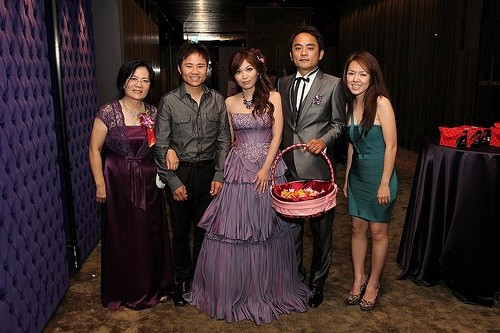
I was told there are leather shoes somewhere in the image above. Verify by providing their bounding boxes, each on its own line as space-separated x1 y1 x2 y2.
307 284 324 308
173 280 189 307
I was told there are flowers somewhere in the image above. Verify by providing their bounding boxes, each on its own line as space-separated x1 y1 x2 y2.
138 110 156 128
312 96 322 105
248 48 268 64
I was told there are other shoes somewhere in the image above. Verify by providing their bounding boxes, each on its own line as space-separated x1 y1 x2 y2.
159 295 169 303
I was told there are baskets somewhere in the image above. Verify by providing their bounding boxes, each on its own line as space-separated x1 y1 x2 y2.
270 143 337 218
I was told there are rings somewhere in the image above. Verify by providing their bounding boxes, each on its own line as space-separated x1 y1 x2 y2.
313 148 317 151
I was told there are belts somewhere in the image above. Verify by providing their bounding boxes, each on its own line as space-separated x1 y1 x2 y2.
178 160 210 169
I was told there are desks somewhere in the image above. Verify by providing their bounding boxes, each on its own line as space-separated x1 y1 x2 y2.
396 133 500 308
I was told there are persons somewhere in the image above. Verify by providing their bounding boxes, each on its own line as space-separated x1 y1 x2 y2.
276 27 345 310
89 59 179 310
155 44 231 306
182 49 315 326
343 50 399 310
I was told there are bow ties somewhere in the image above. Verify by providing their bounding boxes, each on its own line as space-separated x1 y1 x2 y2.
295 67 320 111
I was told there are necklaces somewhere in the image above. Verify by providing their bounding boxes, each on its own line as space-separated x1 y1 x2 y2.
241 91 255 109
122 99 145 127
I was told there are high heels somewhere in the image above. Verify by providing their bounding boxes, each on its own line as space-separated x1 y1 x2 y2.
346 273 368 304
360 279 380 311
